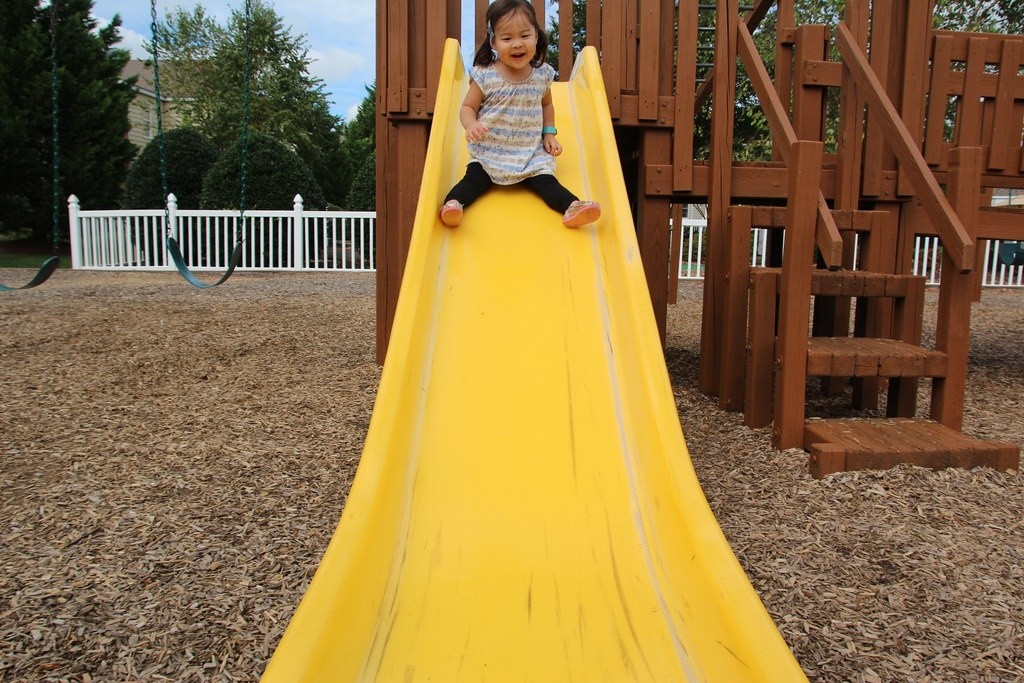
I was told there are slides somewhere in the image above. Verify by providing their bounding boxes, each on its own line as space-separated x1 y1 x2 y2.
257 37 815 679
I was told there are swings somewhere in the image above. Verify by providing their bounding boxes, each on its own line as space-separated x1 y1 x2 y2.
0 0 64 292
149 0 252 291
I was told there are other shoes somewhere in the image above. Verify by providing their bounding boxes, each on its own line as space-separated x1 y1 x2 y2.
563 201 601 228
439 199 462 227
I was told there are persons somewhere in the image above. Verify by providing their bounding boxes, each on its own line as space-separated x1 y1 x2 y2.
441 0 601 228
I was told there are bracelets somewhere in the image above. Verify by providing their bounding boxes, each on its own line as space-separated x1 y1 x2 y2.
543 127 558 136
